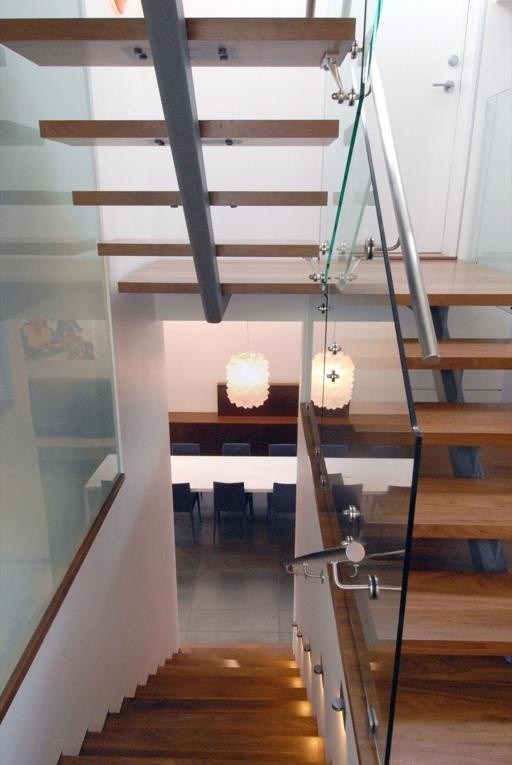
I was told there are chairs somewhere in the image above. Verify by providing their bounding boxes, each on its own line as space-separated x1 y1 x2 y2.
328 472 363 538
172 443 296 546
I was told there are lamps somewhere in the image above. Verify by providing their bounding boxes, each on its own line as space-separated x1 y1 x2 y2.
225 321 270 410
311 322 356 411
